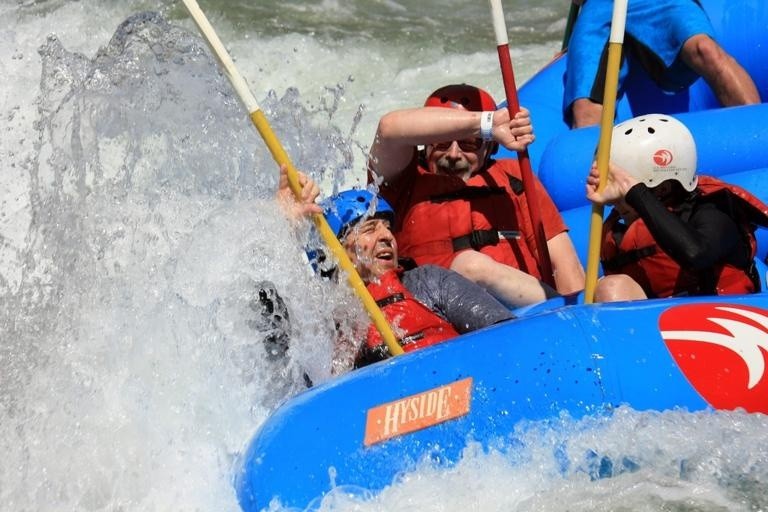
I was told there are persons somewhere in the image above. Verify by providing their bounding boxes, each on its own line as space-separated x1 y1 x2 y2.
563 0 762 130
585 113 768 304
368 84 586 311
259 165 517 388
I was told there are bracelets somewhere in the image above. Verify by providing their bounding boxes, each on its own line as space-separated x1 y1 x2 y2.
480 111 494 142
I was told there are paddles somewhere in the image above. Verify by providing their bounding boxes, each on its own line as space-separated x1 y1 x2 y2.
184 0 405 356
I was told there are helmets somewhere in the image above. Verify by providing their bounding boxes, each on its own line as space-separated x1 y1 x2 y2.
610 113 699 191
424 84 500 156
306 189 394 276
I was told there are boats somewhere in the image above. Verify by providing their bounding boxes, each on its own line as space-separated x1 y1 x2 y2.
233 0 768 512
